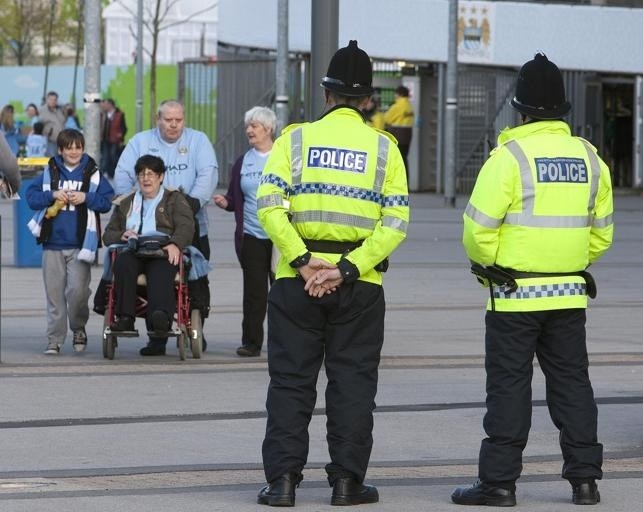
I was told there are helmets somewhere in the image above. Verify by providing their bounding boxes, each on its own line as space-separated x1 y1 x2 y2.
509 52 573 119
319 40 374 97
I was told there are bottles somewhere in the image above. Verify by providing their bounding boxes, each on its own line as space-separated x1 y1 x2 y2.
44 197 65 220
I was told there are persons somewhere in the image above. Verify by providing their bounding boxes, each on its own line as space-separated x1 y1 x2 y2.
102 153 196 333
383 85 415 194
212 106 279 357
0 91 128 201
450 50 614 508
364 94 384 130
24 129 115 355
254 39 410 506
112 98 219 357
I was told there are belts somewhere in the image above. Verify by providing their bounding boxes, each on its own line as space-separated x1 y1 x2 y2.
502 269 582 279
302 239 356 253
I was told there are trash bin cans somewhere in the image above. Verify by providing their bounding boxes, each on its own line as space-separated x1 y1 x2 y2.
10 171 45 268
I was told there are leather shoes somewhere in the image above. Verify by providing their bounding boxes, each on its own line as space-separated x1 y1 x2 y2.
112 320 167 356
572 481 600 504
237 344 260 356
331 478 378 505
258 477 295 506
452 480 516 506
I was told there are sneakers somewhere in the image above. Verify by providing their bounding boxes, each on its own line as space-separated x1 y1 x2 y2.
74 329 86 352
45 345 60 354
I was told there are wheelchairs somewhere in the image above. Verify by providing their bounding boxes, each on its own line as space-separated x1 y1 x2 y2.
96 238 207 362
